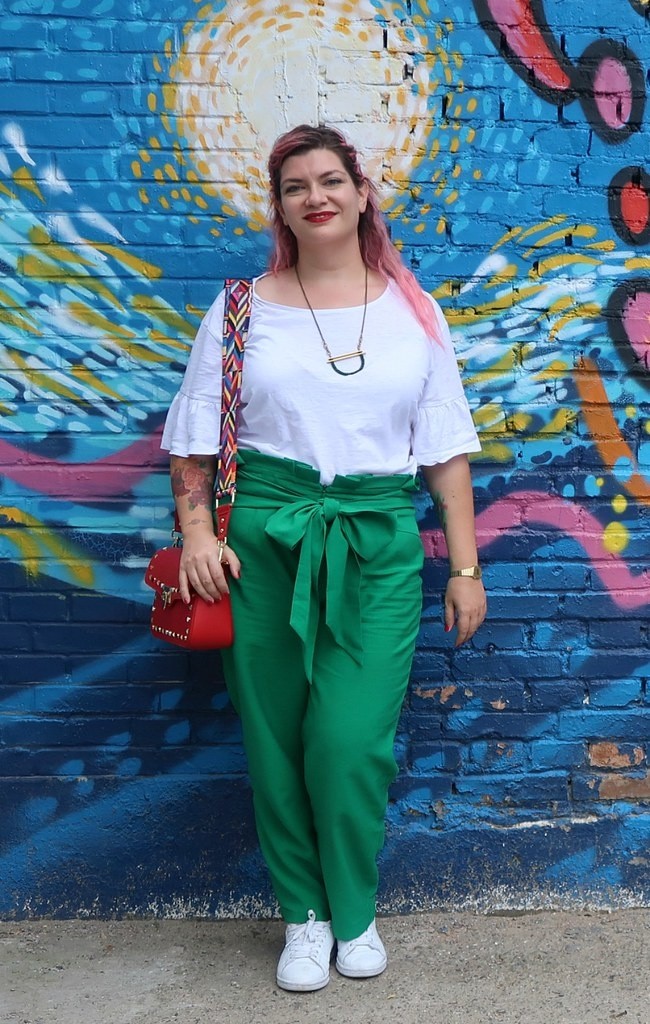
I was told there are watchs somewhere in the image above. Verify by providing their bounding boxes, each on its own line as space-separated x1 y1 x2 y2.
449 565 482 579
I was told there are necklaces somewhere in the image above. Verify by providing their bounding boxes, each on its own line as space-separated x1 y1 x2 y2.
293 257 368 377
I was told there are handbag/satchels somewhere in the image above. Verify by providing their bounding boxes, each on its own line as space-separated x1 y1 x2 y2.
144 545 233 649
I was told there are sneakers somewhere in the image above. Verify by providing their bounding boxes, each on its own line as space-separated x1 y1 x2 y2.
335 917 387 977
276 908 335 992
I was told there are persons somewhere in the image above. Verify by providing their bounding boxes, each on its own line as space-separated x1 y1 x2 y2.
167 126 489 991
